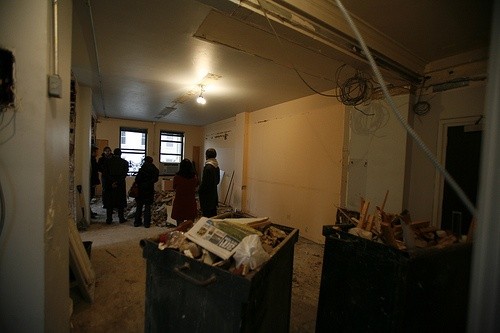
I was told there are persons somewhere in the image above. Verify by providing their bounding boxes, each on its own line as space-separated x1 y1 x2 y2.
98 147 114 208
198 148 220 218
171 159 200 227
106 149 129 224
90 147 101 219
134 156 159 228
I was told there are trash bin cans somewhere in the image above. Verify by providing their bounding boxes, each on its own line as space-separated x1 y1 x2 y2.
314 190 472 333
140 211 299 333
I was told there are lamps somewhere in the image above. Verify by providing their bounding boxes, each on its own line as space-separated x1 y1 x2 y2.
196 86 206 104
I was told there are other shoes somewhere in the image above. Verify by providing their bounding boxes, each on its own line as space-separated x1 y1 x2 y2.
144 223 151 228
106 220 112 224
134 223 143 227
120 219 127 223
91 213 97 219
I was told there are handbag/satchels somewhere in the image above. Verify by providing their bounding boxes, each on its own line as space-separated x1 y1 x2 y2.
129 182 136 197
